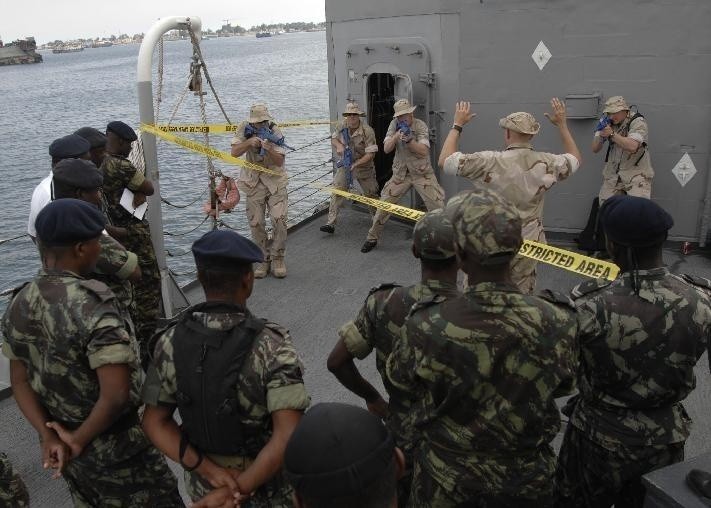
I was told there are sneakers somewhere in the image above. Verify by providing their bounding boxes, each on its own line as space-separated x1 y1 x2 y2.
270 256 287 277
361 239 378 253
254 259 273 278
320 224 336 233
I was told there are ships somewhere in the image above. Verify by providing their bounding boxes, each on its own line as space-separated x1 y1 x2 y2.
0 37 113 65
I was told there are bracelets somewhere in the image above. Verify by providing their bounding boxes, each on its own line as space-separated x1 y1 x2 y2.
451 125 462 133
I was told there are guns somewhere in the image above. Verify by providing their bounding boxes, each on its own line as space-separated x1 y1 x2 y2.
244 124 296 156
396 120 410 136
336 128 353 195
594 117 610 133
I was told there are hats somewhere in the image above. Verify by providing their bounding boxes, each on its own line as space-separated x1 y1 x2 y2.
191 230 265 271
413 188 523 265
500 112 542 136
342 102 366 117
603 95 631 113
246 105 274 124
598 195 674 248
282 404 397 499
35 122 138 245
393 99 417 118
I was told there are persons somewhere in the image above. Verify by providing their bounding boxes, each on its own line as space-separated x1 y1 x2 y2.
231 102 289 279
385 187 579 507
1 197 182 507
29 121 163 326
361 99 444 251
437 97 582 296
327 207 464 508
285 403 406 508
319 104 379 234
553 194 710 508
140 229 312 507
593 96 655 206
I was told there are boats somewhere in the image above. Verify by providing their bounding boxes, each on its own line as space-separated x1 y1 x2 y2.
255 30 272 37
